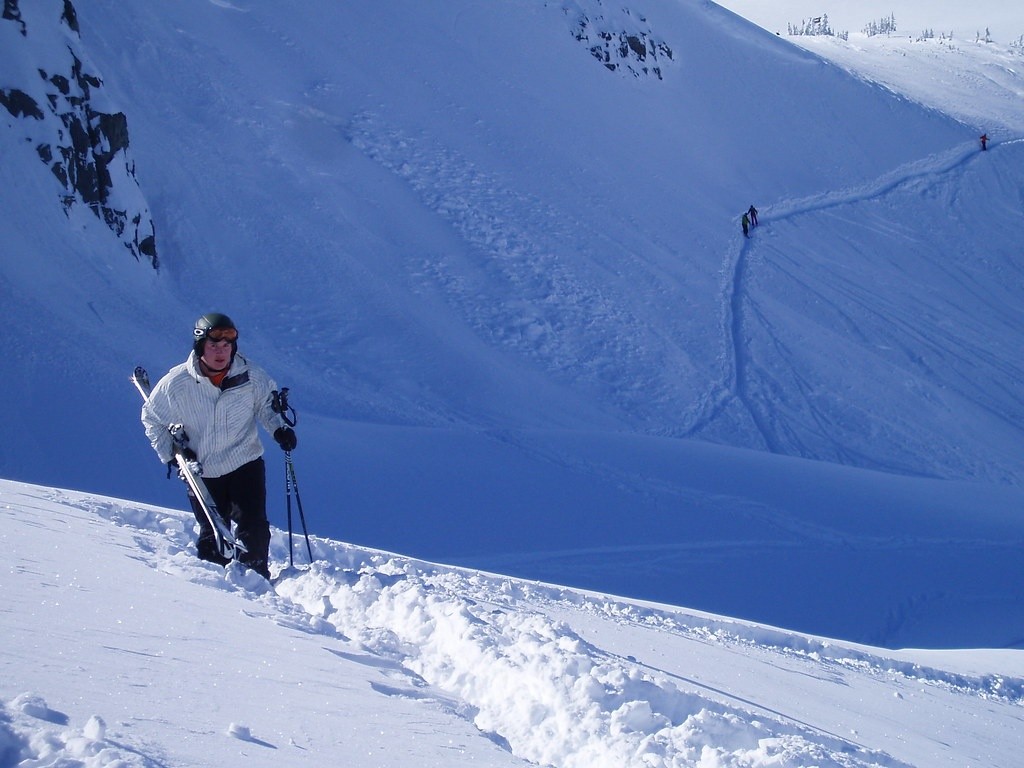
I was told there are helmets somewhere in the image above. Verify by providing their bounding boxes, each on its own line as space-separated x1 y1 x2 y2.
193 312 238 356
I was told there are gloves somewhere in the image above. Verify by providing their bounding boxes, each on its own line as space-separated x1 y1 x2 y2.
170 448 202 478
274 425 298 451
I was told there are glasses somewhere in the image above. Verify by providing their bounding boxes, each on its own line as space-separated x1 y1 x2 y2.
206 326 240 343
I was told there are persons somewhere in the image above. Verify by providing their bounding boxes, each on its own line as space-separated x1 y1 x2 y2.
742 205 758 236
980 134 990 150
141 314 297 579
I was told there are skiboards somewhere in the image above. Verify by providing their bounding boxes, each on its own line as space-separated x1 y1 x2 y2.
129 366 249 559
